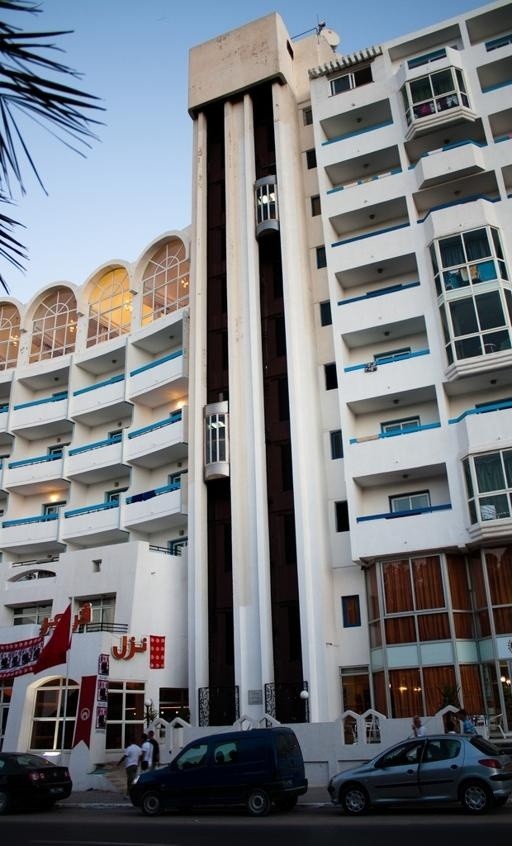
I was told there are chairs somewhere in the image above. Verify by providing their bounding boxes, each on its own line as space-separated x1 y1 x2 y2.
484 714 507 740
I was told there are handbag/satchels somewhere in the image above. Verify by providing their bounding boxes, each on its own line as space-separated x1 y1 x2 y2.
141 760 149 771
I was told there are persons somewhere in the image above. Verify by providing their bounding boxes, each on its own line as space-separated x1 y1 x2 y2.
447 721 457 733
117 730 160 800
413 715 424 736
456 710 476 734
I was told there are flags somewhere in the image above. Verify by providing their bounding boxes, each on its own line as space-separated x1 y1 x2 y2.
34 603 73 675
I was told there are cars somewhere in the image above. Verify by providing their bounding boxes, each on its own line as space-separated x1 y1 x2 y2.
0 752 72 816
327 734 512 816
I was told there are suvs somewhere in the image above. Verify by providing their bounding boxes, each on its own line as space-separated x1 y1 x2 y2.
128 727 308 816
